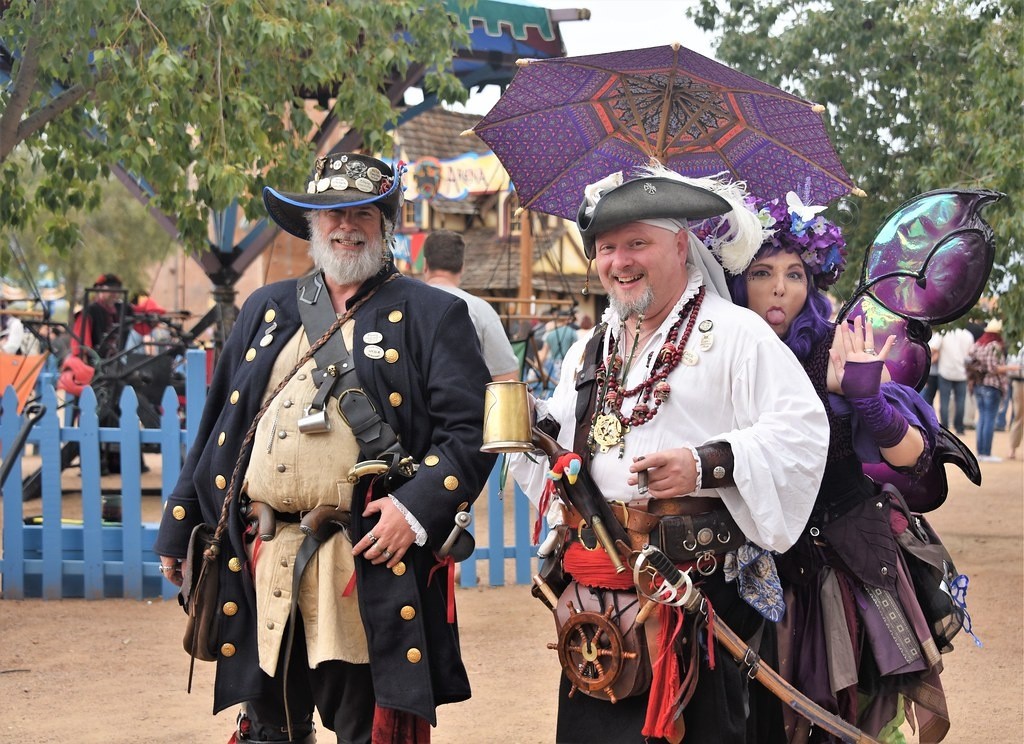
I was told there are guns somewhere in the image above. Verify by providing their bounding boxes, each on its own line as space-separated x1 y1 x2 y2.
530 426 625 575
299 505 351 543
238 502 277 542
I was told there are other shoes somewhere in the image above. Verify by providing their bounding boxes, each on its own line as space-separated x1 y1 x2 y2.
1008 449 1015 459
956 430 964 436
963 425 976 430
994 425 1006 431
978 455 1002 462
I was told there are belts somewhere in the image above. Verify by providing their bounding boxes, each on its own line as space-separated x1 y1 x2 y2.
272 510 343 742
560 497 753 564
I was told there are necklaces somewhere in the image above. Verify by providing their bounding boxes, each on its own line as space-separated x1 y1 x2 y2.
626 323 664 353
587 282 706 459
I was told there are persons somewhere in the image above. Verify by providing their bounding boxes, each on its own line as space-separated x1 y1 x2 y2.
938 327 974 432
419 229 520 383
537 313 579 393
155 151 499 744
919 328 942 403
498 152 830 744
970 314 1020 461
1008 345 1024 456
71 272 136 474
576 314 593 337
694 188 1005 744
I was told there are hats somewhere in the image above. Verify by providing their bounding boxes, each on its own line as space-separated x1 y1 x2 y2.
93 275 122 288
576 177 733 260
263 152 407 240
984 319 1004 333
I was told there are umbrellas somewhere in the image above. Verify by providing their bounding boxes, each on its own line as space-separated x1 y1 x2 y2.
460 41 868 253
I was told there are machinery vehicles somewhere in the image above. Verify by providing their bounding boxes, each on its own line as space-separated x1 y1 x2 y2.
1 286 218 479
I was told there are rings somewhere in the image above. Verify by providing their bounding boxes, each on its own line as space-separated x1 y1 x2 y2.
383 550 392 559
391 556 400 565
374 542 384 554
367 532 377 543
864 348 875 354
158 565 175 574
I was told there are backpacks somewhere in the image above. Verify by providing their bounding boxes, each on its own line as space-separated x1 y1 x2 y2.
965 344 996 390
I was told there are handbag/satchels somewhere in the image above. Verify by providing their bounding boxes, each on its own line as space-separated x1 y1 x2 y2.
896 512 961 621
177 524 225 661
554 582 650 699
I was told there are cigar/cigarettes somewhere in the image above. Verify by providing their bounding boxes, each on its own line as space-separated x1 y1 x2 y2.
637 456 648 495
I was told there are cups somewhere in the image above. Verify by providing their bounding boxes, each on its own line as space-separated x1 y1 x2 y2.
479 380 537 455
